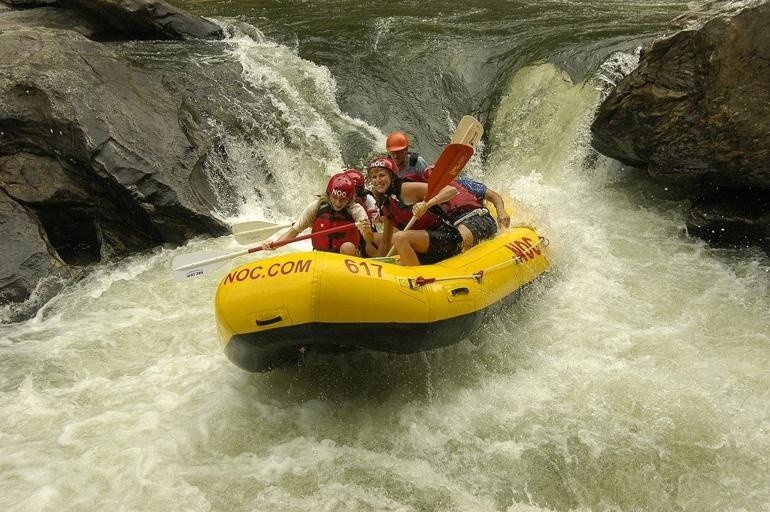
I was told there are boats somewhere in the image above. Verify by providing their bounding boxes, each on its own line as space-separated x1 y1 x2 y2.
214 194 551 374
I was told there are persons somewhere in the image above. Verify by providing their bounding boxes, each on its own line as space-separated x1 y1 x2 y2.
424 164 510 253
372 133 429 202
368 159 464 267
262 174 374 258
343 168 382 256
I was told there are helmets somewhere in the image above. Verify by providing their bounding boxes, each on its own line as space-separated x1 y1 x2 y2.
386 134 408 152
368 157 399 176
424 165 436 179
326 170 364 200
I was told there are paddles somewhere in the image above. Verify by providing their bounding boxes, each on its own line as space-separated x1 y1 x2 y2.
232 214 376 245
451 115 484 148
385 143 473 258
172 221 362 282
